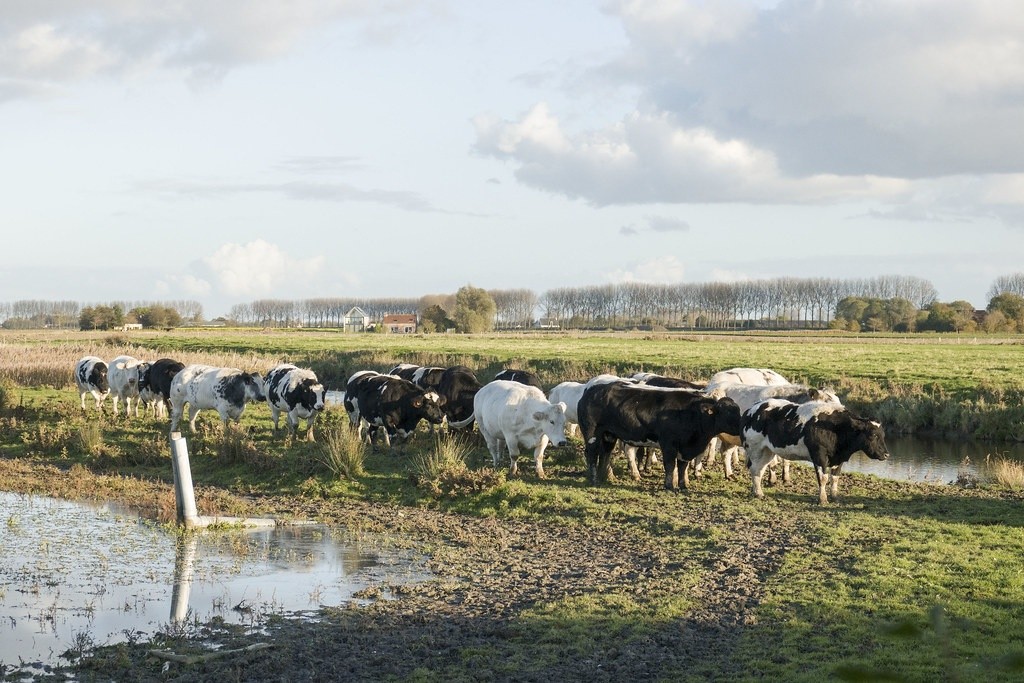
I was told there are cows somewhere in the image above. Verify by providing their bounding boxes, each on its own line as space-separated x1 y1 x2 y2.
75 355 891 506
